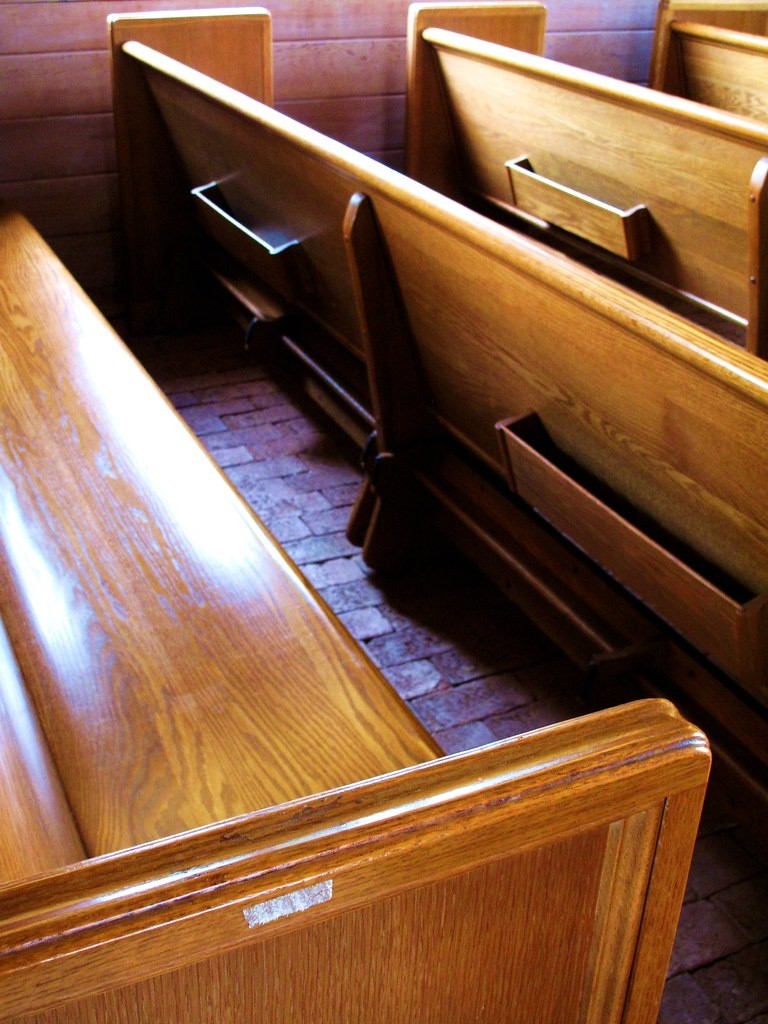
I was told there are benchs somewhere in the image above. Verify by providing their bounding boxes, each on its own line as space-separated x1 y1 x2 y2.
103 7 768 853
647 0 768 124
0 215 715 1024
405 2 768 363
